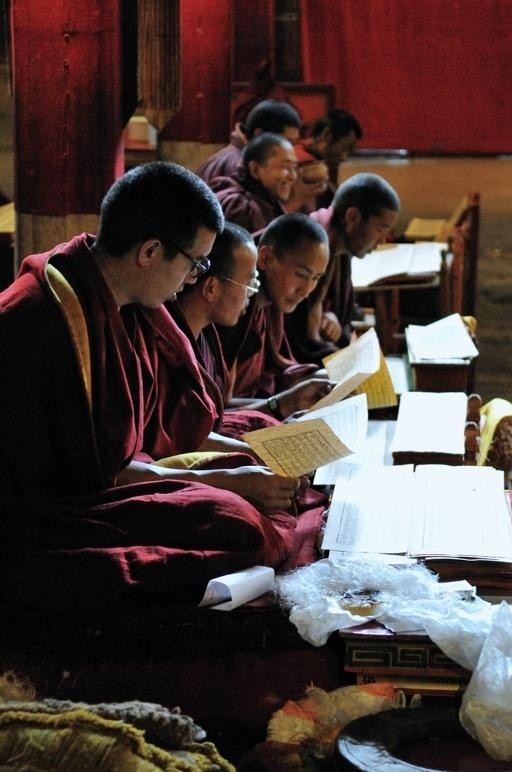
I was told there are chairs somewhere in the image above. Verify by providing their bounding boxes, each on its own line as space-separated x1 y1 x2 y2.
399 190 480 392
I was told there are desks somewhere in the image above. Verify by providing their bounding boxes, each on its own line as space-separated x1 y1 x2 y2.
351 241 439 352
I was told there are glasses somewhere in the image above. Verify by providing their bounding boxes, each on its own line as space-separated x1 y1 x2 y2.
180 250 211 279
224 270 261 298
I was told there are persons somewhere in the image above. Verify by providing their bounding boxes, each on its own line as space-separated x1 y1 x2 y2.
166 171 401 452
1 162 301 628
194 99 364 234
